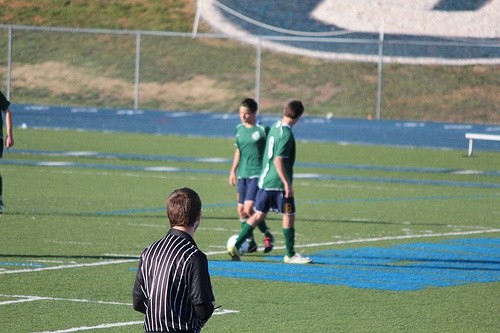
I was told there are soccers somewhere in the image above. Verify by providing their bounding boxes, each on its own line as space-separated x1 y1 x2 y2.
227 234 249 255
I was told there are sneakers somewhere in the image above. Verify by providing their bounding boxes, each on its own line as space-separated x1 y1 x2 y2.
228 245 240 260
263 236 273 253
284 249 312 264
239 240 257 253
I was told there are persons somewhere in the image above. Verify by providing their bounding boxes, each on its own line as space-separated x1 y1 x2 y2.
0 89 15 215
132 187 215 333
226 100 313 264
229 98 274 254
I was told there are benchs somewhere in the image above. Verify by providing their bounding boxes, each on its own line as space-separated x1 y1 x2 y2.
465 133 500 157
